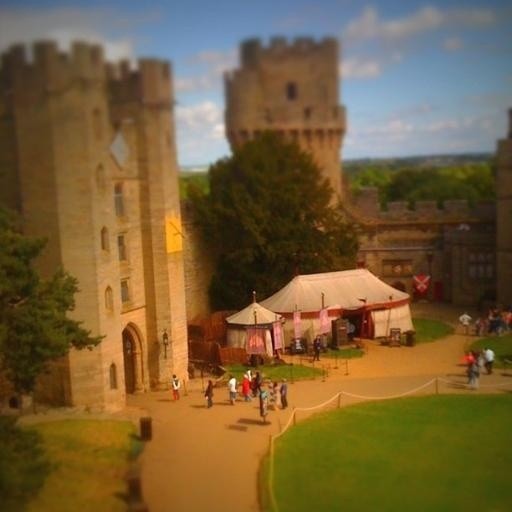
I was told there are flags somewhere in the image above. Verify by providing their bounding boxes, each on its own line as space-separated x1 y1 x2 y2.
292 309 302 338
318 305 332 334
270 320 284 349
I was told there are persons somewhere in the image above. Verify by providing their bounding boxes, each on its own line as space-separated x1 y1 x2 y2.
483 347 495 374
458 311 473 334
243 375 252 401
465 349 483 389
228 375 237 405
475 306 512 336
267 378 287 409
313 335 321 360
205 380 213 408
172 374 181 401
253 372 263 397
247 369 253 387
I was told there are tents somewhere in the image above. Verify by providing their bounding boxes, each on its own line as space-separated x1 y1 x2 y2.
225 291 287 363
258 268 417 352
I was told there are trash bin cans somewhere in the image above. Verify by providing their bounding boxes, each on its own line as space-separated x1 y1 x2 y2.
251 354 259 367
127 475 141 501
140 417 152 441
406 330 416 346
